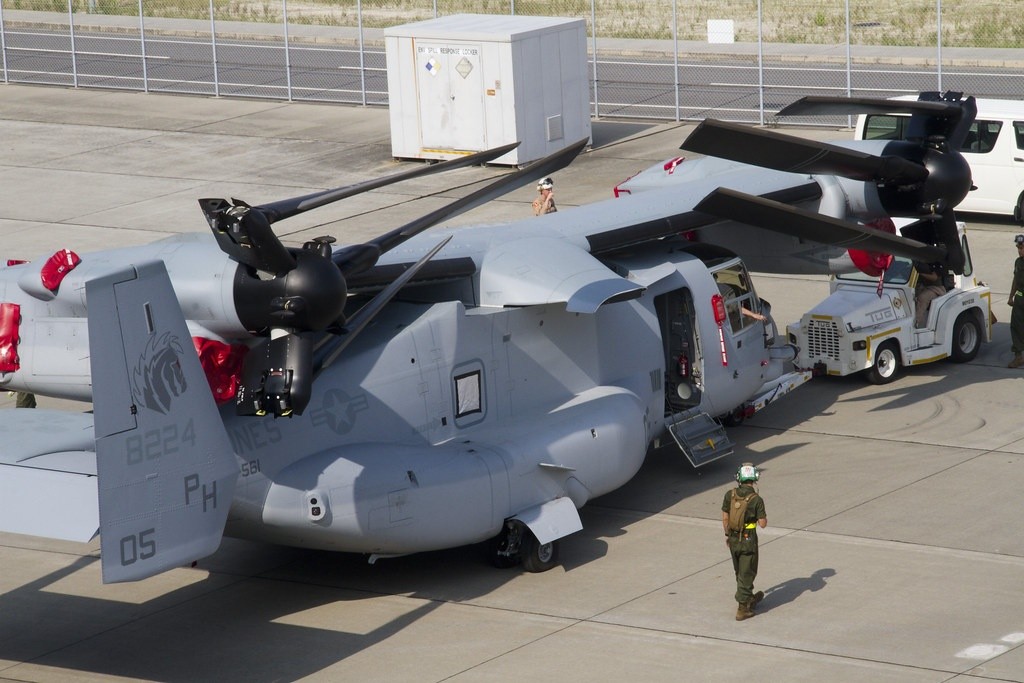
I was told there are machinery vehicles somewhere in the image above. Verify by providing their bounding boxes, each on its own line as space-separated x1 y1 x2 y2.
785 222 996 387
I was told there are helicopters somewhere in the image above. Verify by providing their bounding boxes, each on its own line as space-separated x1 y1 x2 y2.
607 81 979 273
0 135 775 585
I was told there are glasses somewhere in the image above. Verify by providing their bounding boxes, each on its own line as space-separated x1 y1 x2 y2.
542 178 553 185
1014 235 1024 243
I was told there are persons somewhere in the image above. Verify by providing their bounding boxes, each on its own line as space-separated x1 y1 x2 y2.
721 463 768 622
1008 235 1024 369
914 262 947 327
711 273 768 331
532 177 558 216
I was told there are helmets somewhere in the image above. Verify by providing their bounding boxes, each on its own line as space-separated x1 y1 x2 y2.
537 178 553 190
735 462 760 484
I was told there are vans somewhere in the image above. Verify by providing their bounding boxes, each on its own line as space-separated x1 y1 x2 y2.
854 94 1024 225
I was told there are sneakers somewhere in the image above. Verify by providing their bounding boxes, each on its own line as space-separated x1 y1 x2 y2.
1008 356 1024 368
736 608 756 620
751 591 764 609
